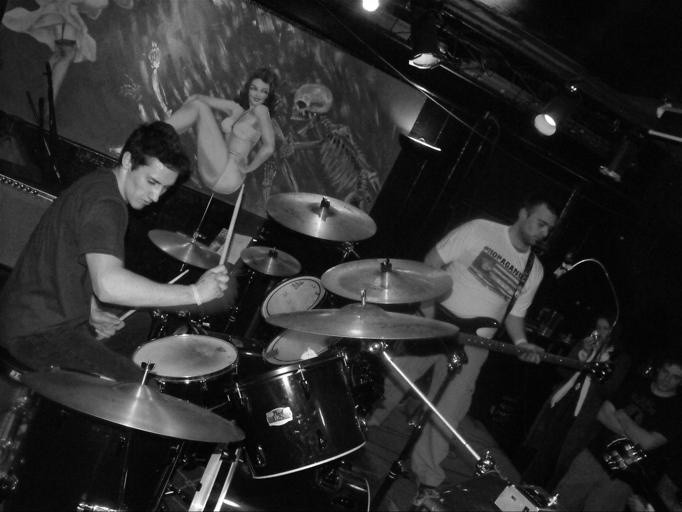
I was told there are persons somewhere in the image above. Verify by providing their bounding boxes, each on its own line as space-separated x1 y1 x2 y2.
1 119 234 391
156 70 276 196
350 188 565 512
514 312 635 494
3 1 136 128
540 348 682 512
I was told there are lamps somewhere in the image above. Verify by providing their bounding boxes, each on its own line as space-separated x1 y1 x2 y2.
599 143 636 183
530 97 572 137
406 15 444 72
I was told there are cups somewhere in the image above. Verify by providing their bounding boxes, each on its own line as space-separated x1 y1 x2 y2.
536 308 565 337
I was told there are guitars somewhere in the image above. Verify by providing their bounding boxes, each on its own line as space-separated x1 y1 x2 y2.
369 299 607 384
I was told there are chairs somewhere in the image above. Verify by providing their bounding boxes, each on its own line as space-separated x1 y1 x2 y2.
1 263 35 491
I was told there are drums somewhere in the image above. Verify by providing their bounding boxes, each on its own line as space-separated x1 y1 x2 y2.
234 350 366 478
262 329 346 364
262 277 325 324
133 333 235 415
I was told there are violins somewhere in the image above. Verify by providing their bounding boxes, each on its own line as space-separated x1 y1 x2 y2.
22 63 77 190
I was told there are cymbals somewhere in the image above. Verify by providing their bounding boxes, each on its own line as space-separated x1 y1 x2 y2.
11 366 244 450
149 230 220 272
322 258 451 304
265 190 378 247
267 290 459 350
238 245 301 278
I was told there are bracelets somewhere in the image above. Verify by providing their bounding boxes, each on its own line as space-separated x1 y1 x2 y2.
189 283 203 308
